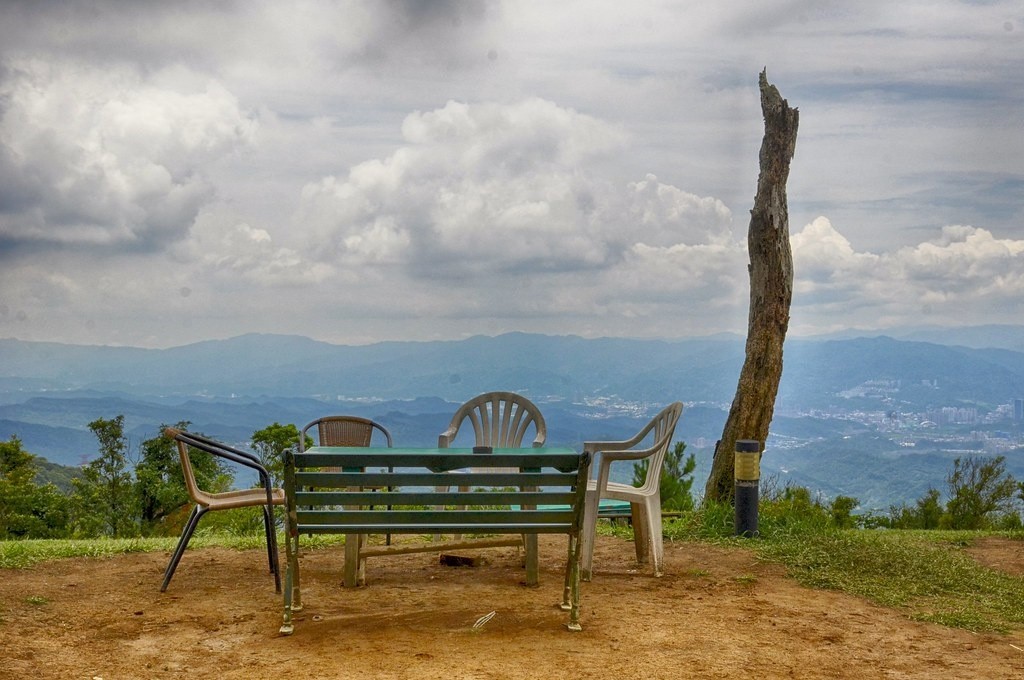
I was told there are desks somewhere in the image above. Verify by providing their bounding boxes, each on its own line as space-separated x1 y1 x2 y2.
303 446 577 586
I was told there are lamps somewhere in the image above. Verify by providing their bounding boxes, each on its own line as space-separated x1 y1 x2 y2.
734 439 760 486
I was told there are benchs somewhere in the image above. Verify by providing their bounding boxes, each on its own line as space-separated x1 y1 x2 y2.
282 450 592 632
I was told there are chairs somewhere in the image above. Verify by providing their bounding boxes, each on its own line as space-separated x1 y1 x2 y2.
297 415 393 545
579 403 683 581
432 392 546 541
159 427 286 595
